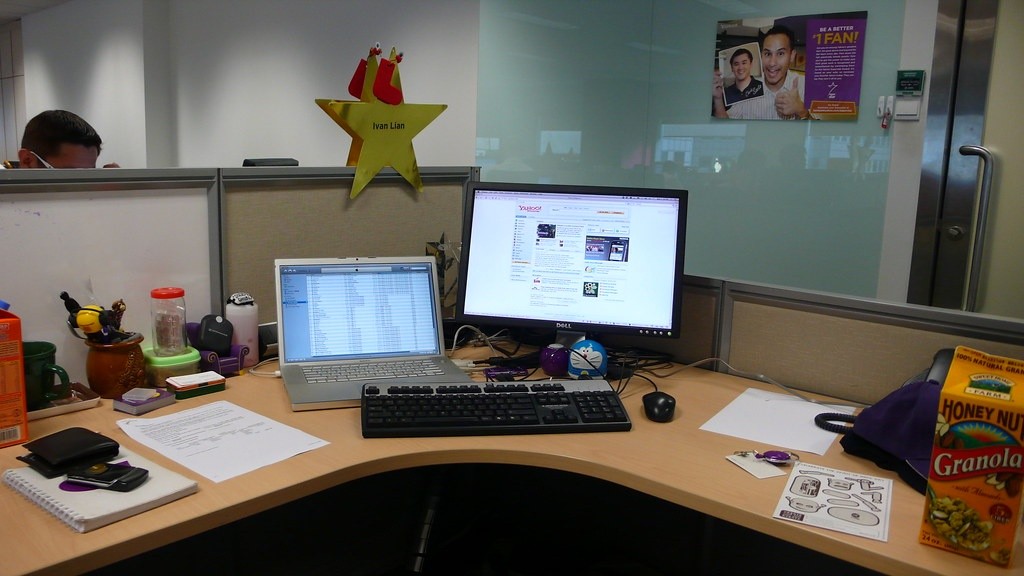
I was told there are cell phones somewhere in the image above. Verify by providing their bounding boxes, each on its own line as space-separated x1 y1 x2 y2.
67 461 149 494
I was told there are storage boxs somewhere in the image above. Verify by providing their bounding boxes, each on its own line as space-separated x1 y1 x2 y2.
138 346 201 392
918 345 1023 568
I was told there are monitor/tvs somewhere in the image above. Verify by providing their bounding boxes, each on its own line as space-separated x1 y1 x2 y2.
456 180 689 380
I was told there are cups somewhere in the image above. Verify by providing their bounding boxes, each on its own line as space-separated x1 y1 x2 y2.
21 341 69 411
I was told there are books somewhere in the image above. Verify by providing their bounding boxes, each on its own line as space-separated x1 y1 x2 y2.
1 442 199 536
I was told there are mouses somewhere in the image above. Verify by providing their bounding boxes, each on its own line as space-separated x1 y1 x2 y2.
642 391 676 423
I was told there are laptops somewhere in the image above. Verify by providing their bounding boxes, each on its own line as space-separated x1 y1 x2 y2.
275 255 474 413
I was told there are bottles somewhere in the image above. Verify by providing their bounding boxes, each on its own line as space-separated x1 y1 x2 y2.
149 287 188 355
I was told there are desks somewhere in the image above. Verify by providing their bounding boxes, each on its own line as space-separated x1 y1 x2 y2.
0 334 1024 575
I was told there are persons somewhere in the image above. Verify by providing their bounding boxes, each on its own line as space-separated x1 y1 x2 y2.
724 48 764 105
0 110 122 169
713 26 809 120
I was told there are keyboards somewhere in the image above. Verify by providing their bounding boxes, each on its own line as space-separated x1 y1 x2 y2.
360 380 632 439
